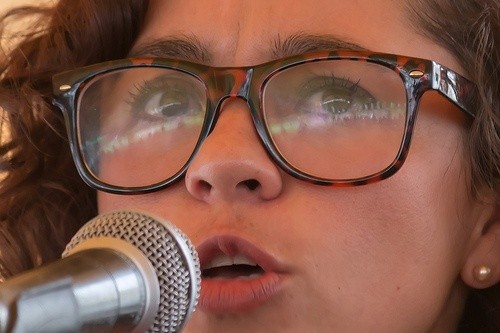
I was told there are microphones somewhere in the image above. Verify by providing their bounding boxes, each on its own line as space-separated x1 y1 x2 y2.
0 208 201 333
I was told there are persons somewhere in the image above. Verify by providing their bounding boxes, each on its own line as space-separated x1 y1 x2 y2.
1 1 500 333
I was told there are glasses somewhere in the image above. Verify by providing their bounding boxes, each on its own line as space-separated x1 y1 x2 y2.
48 49 480 194
